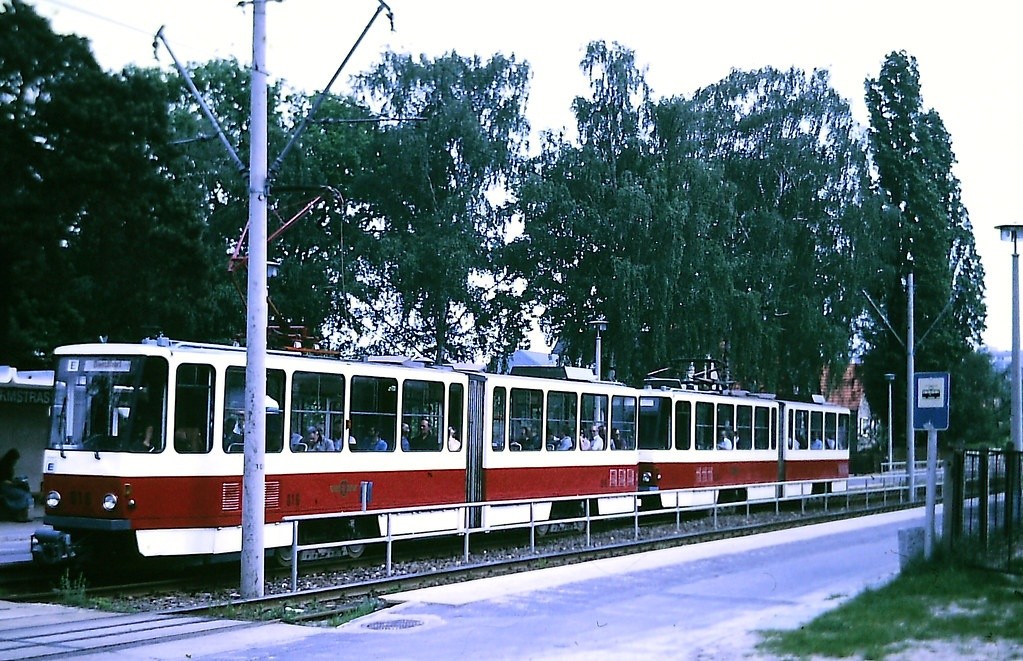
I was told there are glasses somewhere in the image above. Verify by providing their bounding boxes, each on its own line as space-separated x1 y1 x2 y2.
590 429 598 432
420 425 428 427
581 432 584 434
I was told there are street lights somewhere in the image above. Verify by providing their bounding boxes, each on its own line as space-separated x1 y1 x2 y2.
884 373 896 470
994 223 1023 515
590 313 609 432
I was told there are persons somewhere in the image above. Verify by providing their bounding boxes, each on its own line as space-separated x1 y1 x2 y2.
0 448 31 522
708 430 733 450
132 414 461 453
788 431 844 450
513 425 629 450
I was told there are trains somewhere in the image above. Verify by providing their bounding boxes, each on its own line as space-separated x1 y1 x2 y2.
39 330 852 570
